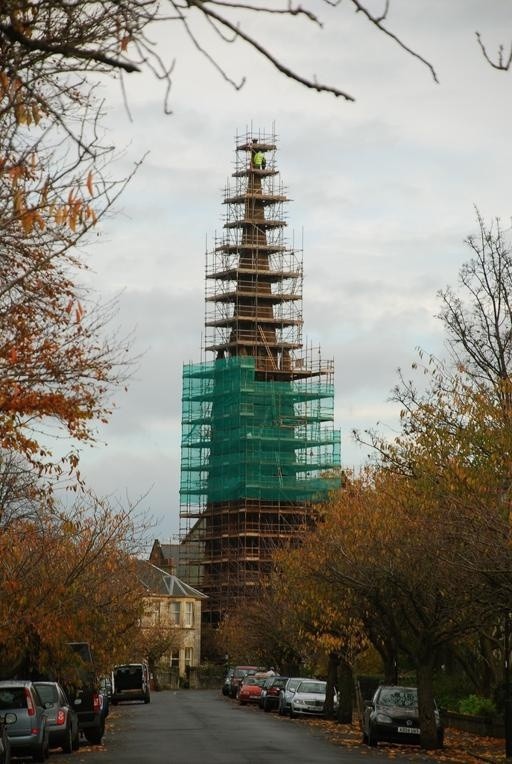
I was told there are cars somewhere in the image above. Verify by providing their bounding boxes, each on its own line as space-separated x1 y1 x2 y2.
222 664 341 718
0 680 55 764
33 680 82 753
360 685 444 750
1 711 18 764
97 672 112 718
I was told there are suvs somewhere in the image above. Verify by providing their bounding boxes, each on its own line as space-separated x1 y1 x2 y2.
58 641 111 745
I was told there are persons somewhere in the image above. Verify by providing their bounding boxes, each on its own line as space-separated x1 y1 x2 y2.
266 666 276 677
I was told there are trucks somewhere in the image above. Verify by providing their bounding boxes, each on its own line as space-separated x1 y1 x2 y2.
111 662 151 706
